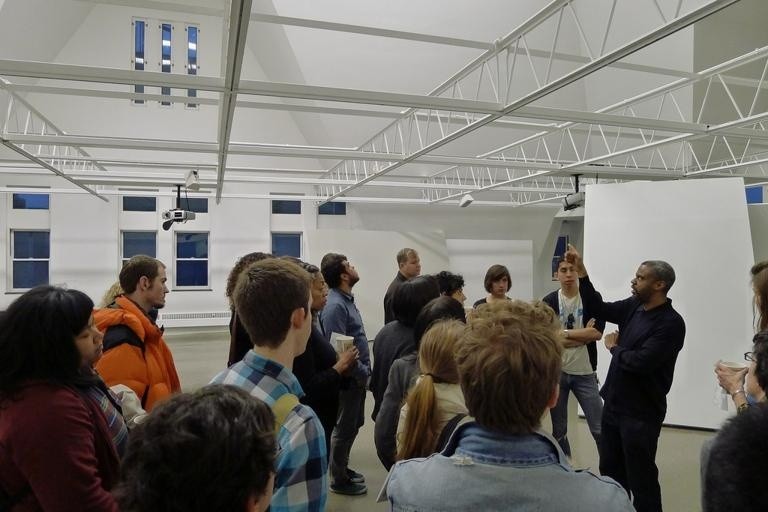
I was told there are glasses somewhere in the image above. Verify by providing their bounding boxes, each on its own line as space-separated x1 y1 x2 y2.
567 314 574 329
744 351 759 363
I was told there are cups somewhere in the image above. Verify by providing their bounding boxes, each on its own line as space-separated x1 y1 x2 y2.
720 362 747 394
336 337 354 354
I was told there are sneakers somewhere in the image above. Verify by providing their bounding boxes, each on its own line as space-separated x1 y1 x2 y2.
346 468 364 482
329 481 368 495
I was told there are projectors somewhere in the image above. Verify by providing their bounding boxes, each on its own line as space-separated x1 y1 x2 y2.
562 192 585 211
162 209 195 230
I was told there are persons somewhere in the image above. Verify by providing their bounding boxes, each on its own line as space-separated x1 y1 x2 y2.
371 244 686 512
699 261 767 512
0 253 372 512
